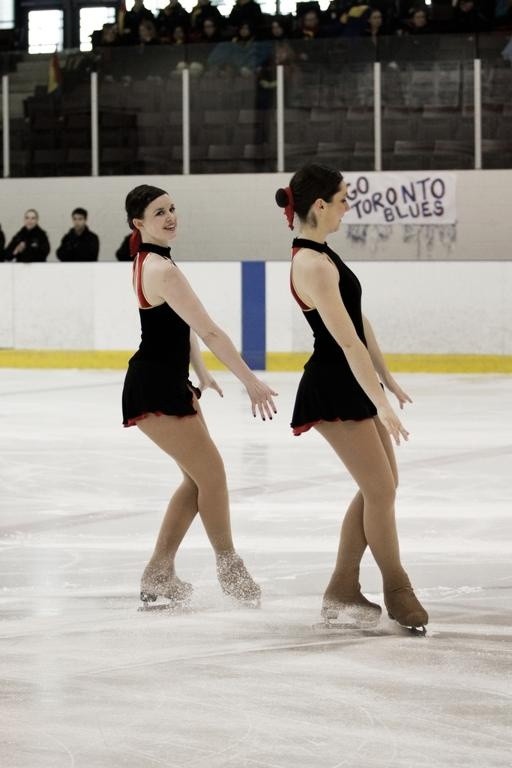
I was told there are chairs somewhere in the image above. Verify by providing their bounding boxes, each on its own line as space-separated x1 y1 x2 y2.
0 35 512 174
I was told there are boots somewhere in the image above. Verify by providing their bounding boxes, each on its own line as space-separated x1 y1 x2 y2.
323 575 381 620
382 583 428 626
216 552 260 602
140 554 193 602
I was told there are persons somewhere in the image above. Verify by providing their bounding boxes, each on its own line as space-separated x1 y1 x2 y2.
112 231 142 262
2 209 52 264
81 1 511 77
52 207 100 263
117 184 282 608
270 161 434 633
